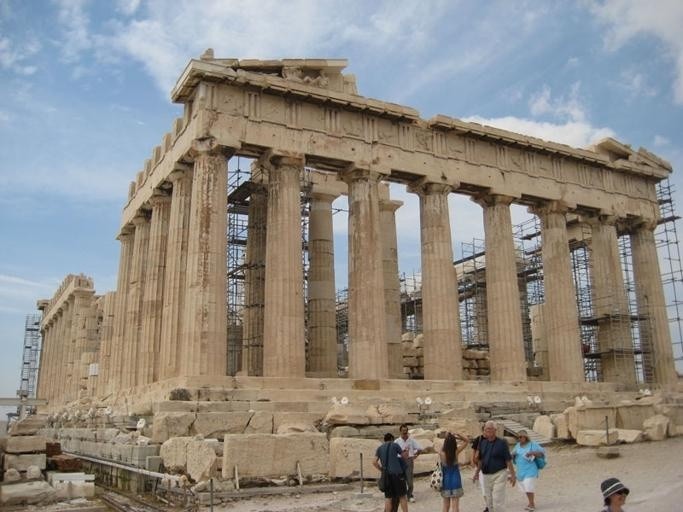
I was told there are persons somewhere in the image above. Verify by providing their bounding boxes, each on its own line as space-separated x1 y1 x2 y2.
471 424 488 512
599 478 629 512
439 431 469 512
372 424 422 512
472 420 516 512
510 430 546 512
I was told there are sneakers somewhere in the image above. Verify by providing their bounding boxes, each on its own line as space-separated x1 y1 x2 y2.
408 497 415 502
524 505 535 512
483 507 489 512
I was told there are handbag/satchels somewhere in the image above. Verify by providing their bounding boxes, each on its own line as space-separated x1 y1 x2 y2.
377 472 392 492
429 469 443 492
535 454 546 469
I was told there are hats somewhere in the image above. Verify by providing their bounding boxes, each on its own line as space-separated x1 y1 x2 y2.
600 476 630 506
